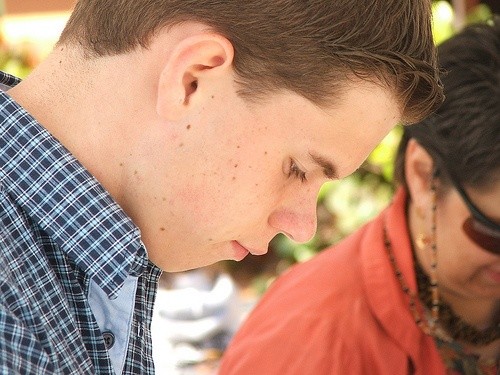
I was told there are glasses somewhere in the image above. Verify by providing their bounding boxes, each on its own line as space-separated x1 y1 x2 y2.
430 141 500 255
